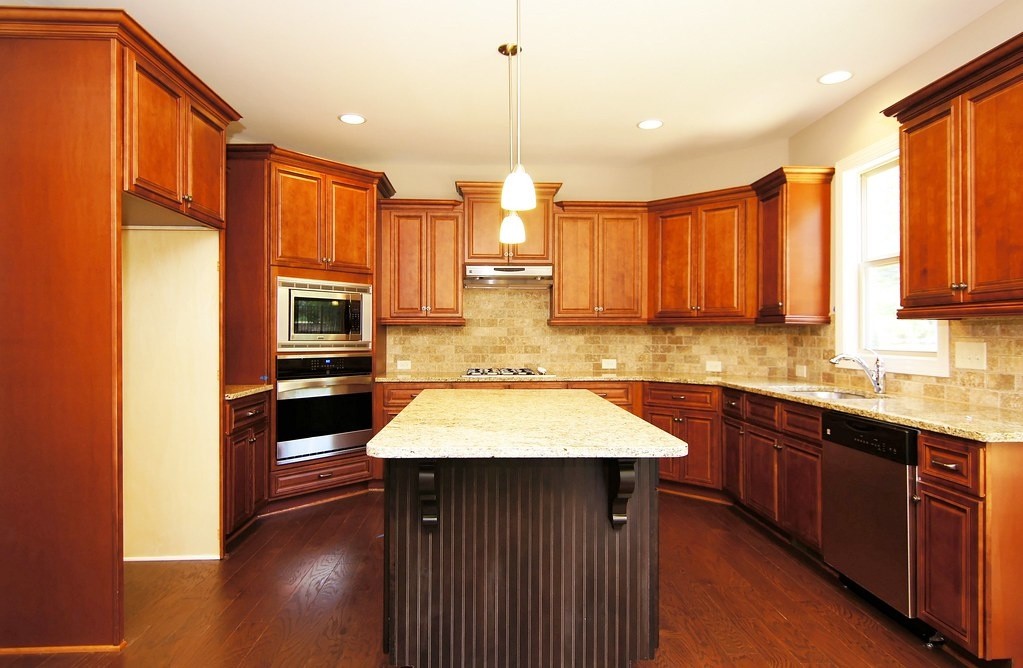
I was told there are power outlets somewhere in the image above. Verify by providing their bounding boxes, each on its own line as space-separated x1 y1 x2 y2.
706 361 722 372
601 359 617 369
955 342 986 369
397 361 412 369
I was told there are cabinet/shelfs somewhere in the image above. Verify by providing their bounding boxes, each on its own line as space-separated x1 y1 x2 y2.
3 2 1022 666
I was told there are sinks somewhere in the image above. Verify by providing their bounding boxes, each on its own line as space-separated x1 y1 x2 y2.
786 389 893 400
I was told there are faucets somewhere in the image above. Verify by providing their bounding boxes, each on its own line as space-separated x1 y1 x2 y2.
829 352 885 394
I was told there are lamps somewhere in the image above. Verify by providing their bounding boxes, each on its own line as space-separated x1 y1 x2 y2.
499 43 525 243
501 0 537 211
